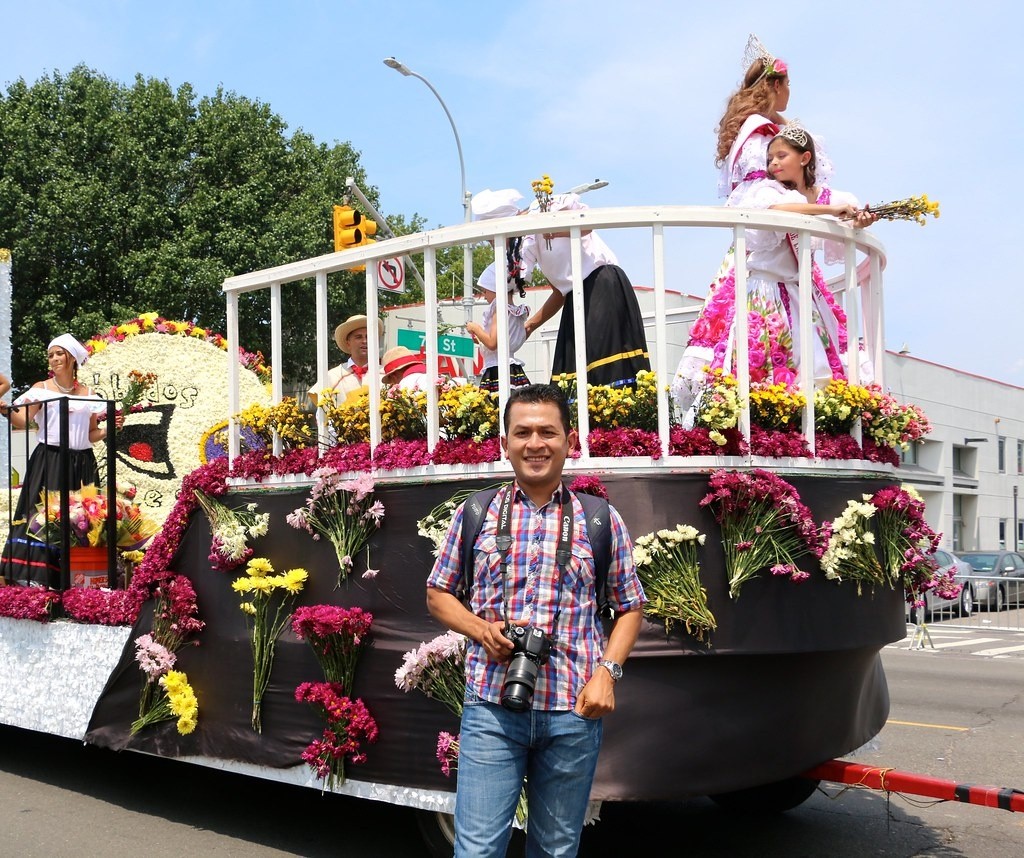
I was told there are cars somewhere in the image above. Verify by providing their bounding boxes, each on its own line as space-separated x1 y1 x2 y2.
904 547 977 623
948 550 1024 612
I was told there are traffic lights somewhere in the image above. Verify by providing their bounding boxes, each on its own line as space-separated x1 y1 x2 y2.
332 205 362 252
359 215 377 270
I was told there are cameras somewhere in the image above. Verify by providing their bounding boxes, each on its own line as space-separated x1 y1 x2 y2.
501 623 552 714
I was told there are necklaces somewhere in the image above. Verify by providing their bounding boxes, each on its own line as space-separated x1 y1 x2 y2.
53 375 75 393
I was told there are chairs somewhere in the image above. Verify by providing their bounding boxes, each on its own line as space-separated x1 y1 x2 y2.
993 558 1005 570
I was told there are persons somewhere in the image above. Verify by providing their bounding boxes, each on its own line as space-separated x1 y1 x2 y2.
427 384 649 858
670 34 880 431
467 261 532 398
471 189 651 399
381 347 456 441
0 333 126 593
0 372 11 398
307 315 391 448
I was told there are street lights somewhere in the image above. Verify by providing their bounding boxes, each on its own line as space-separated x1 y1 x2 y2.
383 58 472 328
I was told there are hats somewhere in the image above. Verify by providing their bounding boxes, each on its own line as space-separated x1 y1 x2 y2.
471 189 524 220
334 314 385 354
47 332 89 368
476 258 516 294
381 346 425 383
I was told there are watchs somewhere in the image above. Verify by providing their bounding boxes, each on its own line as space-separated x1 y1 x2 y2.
600 660 623 681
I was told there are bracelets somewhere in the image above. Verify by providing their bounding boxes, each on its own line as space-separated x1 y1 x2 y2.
2 409 12 417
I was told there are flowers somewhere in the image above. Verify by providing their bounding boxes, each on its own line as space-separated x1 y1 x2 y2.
609 523 718 651
231 557 310 734
285 466 386 592
192 490 272 574
130 571 205 737
838 193 939 226
289 605 379 797
819 485 963 650
416 481 514 558
0 248 228 628
393 630 528 825
532 174 554 252
699 468 832 603
764 59 789 78
229 346 933 484
